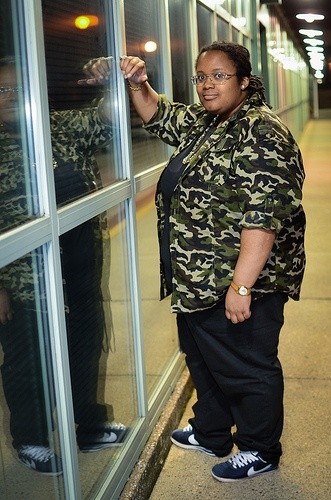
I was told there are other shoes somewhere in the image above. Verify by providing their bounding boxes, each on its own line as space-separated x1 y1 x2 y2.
81 421 131 453
15 444 63 476
170 425 218 457
211 449 280 482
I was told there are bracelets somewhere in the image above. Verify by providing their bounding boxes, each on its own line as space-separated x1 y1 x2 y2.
99 88 112 93
127 82 146 91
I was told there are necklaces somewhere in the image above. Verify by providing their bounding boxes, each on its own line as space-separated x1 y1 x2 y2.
182 114 219 164
32 152 59 170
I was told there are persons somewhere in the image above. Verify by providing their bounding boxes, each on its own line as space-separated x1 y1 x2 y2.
0 55 133 475
120 41 307 483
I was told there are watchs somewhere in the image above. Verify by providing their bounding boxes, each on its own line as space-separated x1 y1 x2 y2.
230 280 251 295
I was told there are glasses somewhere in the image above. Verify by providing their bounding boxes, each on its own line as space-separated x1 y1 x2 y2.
0 85 20 97
189 71 239 86
